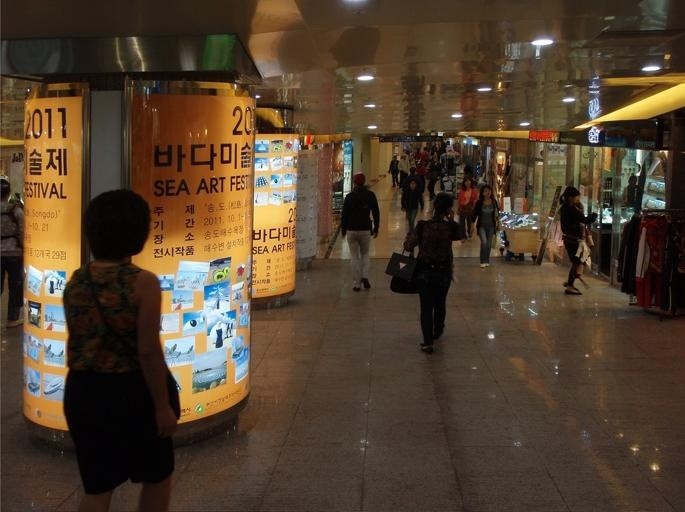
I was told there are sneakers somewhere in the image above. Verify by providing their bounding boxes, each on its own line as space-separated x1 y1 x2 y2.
477 261 492 272
419 326 446 357
353 276 373 294
563 284 582 298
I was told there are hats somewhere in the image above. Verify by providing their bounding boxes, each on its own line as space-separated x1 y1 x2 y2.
350 172 366 186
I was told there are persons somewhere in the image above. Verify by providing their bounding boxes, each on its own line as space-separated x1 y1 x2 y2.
470 185 499 268
404 193 468 354
389 140 480 242
559 186 597 294
62 189 180 512
0 178 25 328
625 176 638 207
341 174 379 292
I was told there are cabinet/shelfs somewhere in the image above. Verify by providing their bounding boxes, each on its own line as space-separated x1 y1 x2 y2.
593 203 634 271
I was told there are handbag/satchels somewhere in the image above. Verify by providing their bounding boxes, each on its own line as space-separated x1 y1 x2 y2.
385 243 422 295
164 357 182 420
